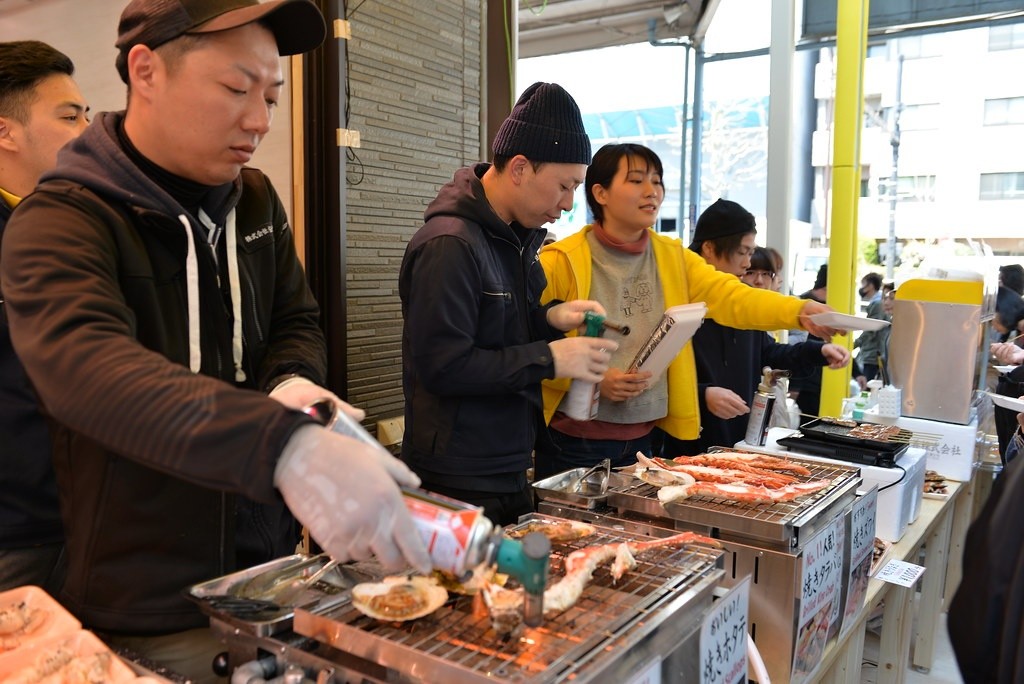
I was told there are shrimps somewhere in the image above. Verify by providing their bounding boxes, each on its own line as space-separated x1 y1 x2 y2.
474 531 723 632
635 451 830 505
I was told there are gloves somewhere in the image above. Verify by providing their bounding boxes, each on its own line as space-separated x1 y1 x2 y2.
272 422 432 575
546 299 606 332
268 377 366 424
548 335 619 383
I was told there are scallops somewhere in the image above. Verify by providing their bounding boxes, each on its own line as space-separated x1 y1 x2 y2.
352 574 449 622
437 519 598 596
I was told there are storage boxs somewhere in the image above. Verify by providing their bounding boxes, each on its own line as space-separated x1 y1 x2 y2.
734 426 927 544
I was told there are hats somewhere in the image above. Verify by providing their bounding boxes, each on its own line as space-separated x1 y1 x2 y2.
492 82 591 165
688 198 755 252
115 0 327 57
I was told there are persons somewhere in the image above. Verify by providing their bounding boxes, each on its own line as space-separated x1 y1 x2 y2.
686 197 853 457
947 265 1024 683
0 0 432 636
0 39 94 595
798 263 867 391
398 82 619 526
536 143 847 482
739 247 792 395
852 272 885 379
876 282 896 385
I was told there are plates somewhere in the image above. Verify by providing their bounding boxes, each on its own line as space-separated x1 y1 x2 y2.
810 312 890 330
986 392 1024 413
640 301 707 375
994 364 1019 374
0 585 160 684
802 418 912 449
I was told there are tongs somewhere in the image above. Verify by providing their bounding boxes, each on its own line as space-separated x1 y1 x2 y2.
626 315 674 374
242 554 338 606
569 459 610 495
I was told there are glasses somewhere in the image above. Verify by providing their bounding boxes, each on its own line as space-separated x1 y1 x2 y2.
740 269 776 285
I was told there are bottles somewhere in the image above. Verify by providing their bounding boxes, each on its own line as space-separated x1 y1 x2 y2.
856 392 868 407
852 403 864 420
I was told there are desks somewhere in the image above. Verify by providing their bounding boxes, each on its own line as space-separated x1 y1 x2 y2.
802 479 976 683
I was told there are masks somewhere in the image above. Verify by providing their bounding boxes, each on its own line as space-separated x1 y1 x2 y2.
859 284 871 298
